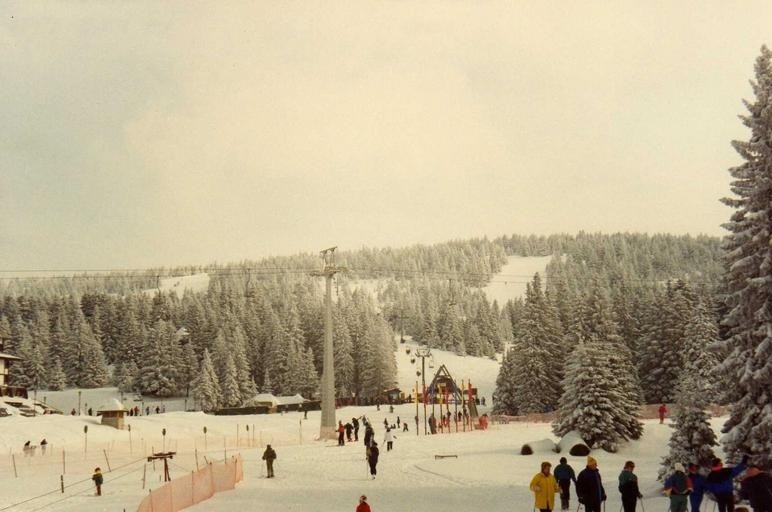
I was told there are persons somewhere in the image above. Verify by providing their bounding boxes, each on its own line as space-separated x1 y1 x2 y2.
529 454 643 512
428 410 488 434
261 443 276 478
357 494 372 512
664 454 772 512
335 415 409 479
21 438 48 457
659 403 666 424
125 406 160 417
94 465 103 496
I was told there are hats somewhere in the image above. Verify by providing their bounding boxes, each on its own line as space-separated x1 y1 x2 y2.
588 456 597 466
